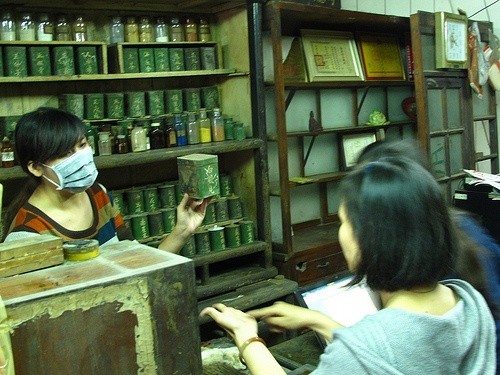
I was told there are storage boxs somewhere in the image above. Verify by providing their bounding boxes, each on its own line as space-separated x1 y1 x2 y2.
0 43 256 259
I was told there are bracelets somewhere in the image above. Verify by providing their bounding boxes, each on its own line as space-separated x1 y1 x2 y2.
239 337 266 366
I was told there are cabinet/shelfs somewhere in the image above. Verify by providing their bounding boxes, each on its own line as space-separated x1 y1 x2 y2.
0 0 276 291
263 2 422 281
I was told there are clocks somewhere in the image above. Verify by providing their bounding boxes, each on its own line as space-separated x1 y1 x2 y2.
432 11 472 69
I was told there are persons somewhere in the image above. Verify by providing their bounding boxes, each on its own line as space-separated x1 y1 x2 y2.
200 156 496 375
357 139 500 375
10 107 212 254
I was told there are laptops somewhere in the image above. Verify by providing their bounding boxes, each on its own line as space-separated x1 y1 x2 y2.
294 270 382 350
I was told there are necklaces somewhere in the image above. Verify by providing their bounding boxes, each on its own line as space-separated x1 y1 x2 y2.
383 282 438 306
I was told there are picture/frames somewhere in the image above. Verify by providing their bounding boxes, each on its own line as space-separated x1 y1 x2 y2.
358 29 409 81
295 29 365 82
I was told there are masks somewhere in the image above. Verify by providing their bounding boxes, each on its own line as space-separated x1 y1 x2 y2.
35 146 99 193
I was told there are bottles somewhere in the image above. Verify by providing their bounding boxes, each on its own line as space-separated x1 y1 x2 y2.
199 108 211 143
139 16 154 43
37 15 54 41
164 122 177 148
124 17 139 43
72 16 87 42
110 17 124 43
98 132 111 156
0 141 15 168
212 107 225 143
186 17 197 41
85 129 96 156
149 122 165 149
199 18 212 42
0 12 17 41
130 122 146 152
116 134 130 155
155 18 170 43
56 16 71 41
187 113 199 144
173 113 187 145
170 17 185 42
17 14 36 41
